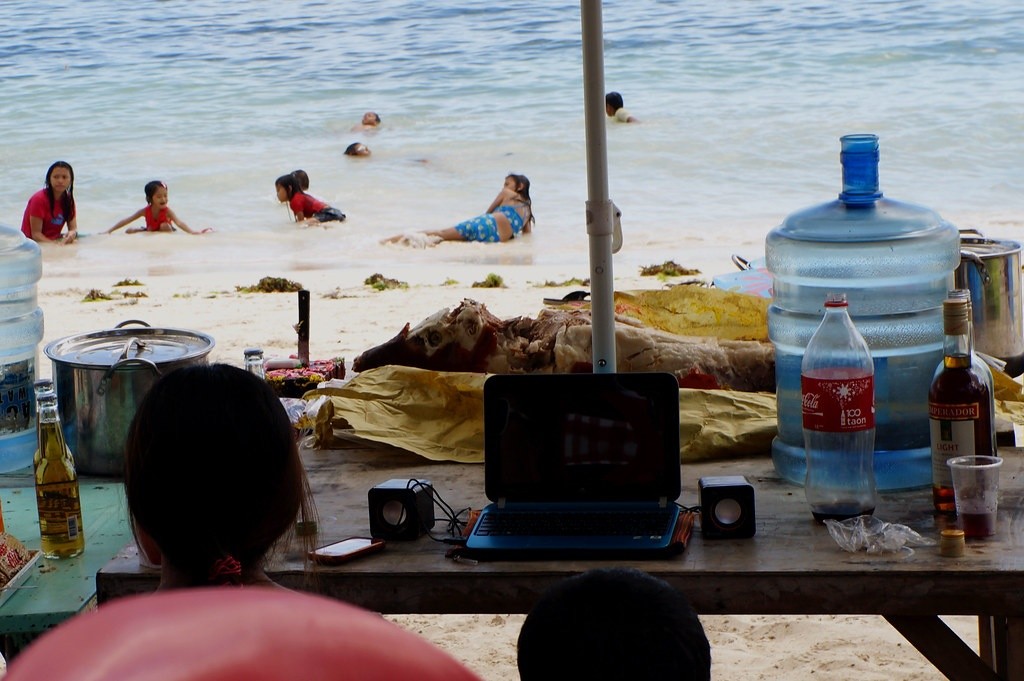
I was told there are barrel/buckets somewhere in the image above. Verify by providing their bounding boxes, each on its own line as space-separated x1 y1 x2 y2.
763 133 963 493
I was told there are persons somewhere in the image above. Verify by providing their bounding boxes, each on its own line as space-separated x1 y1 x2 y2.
275 175 344 226
362 112 380 127
123 364 319 589
291 170 327 204
382 174 534 246
605 92 636 123
345 143 369 155
21 161 77 245
108 181 202 235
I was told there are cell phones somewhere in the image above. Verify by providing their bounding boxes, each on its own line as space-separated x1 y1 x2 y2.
308 537 385 564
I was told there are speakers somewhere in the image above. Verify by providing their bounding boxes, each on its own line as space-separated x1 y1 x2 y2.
368 479 435 541
698 476 756 541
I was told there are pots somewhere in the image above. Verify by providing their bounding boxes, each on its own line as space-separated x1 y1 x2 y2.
44 319 216 477
957 225 1024 379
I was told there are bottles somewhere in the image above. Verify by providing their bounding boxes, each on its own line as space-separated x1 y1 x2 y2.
33 379 76 481
0 218 48 472
802 292 879 524
928 297 996 512
934 296 997 459
242 347 267 381
34 394 85 559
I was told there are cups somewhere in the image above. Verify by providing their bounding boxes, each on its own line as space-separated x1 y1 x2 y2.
945 454 1001 538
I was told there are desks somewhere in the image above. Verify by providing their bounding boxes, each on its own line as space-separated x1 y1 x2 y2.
0 464 135 661
96 439 1024 681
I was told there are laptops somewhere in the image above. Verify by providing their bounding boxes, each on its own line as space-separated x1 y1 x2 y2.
465 374 681 560
